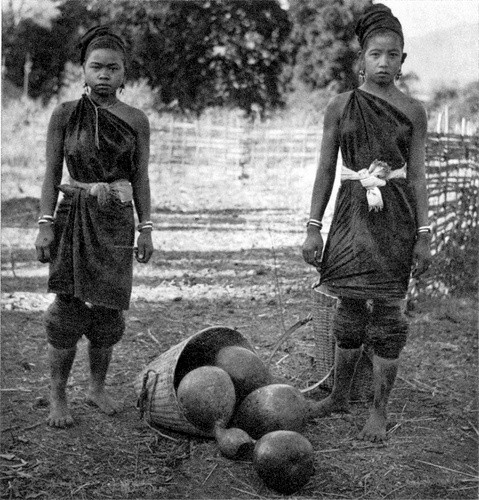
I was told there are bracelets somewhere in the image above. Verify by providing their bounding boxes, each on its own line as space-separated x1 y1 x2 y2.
417 226 431 233
38 215 54 224
309 219 323 230
138 221 152 232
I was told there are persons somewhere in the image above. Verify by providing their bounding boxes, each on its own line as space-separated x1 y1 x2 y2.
302 3 432 441
34 25 154 428
